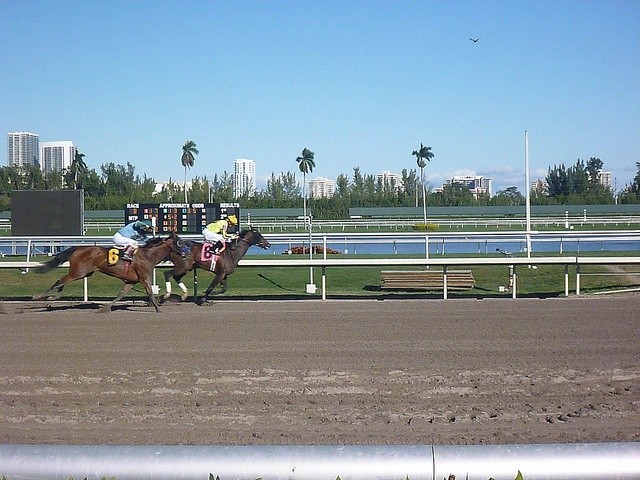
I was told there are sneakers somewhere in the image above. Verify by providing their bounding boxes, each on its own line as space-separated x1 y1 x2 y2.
120 252 133 262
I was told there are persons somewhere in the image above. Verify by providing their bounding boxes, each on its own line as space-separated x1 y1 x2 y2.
113 219 151 261
203 214 240 254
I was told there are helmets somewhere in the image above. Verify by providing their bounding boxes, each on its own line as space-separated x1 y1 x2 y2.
226 215 238 225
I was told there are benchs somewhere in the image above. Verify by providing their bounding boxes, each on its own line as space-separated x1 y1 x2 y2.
380 270 475 290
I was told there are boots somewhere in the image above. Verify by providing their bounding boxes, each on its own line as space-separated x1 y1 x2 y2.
208 241 223 255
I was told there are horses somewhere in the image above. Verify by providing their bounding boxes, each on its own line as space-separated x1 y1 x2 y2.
34 230 194 313
160 226 271 307
